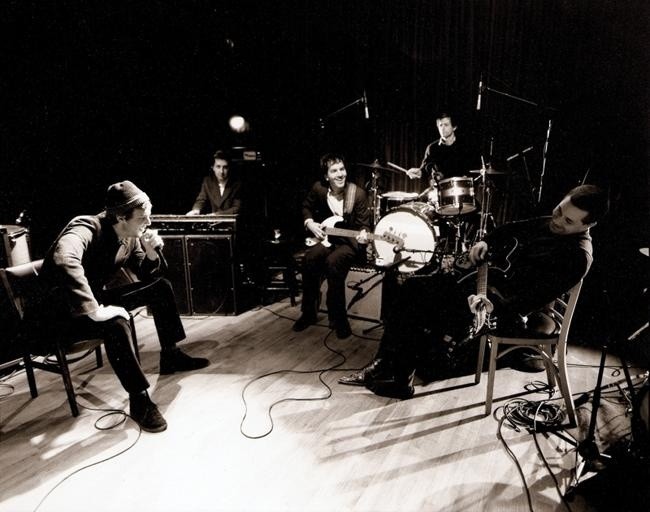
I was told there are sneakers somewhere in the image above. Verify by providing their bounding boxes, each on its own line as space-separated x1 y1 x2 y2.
334 318 352 339
292 313 317 331
130 389 167 433
159 348 210 375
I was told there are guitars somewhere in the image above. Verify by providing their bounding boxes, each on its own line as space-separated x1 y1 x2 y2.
453 234 523 341
304 216 404 248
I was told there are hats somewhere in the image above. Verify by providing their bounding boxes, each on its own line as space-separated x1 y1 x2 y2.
105 180 150 220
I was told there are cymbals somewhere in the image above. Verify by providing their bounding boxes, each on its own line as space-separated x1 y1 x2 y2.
350 161 400 176
470 167 506 175
639 247 650 255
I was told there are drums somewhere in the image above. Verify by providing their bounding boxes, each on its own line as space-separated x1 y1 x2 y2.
372 202 449 274
631 373 650 466
436 176 477 216
380 191 421 218
0 223 32 266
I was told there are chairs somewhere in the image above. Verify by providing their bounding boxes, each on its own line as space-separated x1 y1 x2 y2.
0 256 142 418
474 276 584 424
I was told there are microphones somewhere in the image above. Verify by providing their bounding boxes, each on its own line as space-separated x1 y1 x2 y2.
477 72 483 110
495 146 535 169
363 91 369 119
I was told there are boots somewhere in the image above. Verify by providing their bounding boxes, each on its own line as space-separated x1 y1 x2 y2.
337 343 397 385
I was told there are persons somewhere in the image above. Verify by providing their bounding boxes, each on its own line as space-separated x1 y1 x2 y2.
187 146 242 316
406 109 481 181
334 183 611 402
24 178 211 432
297 152 371 342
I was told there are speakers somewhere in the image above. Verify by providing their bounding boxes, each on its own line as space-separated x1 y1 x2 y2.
184 235 239 317
148 235 190 315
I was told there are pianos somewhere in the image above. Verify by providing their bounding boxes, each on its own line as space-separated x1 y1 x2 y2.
149 214 238 233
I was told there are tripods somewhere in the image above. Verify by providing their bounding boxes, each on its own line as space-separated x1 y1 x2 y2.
531 175 650 505
